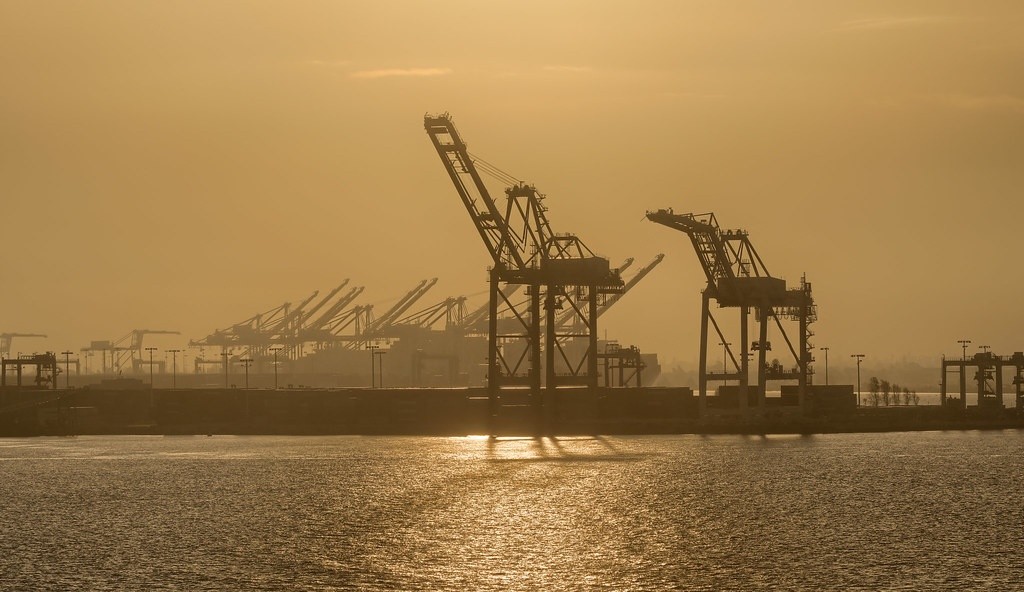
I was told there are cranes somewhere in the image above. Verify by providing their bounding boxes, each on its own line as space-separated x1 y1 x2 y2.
0 253 667 380
637 206 819 409
424 111 625 416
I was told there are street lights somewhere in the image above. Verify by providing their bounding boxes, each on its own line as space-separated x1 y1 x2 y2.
366 346 380 389
168 350 181 388
240 358 254 390
61 350 73 388
851 355 865 406
145 348 157 389
820 347 829 385
719 343 732 387
221 353 233 388
958 340 971 408
269 348 282 390
